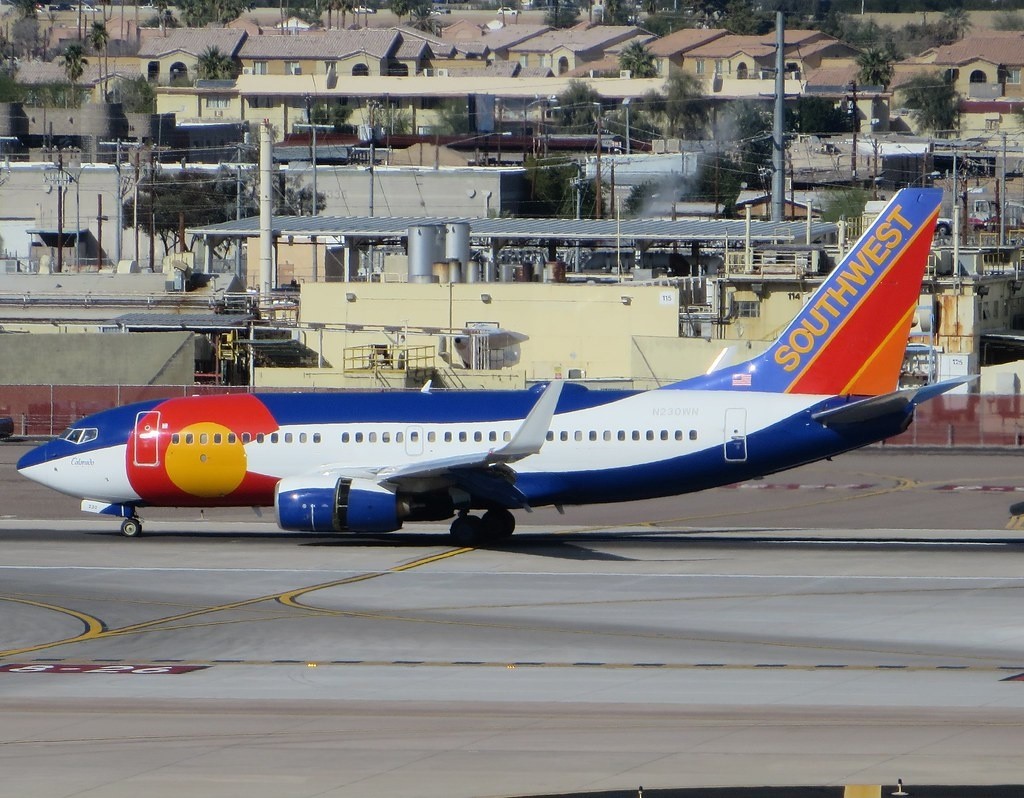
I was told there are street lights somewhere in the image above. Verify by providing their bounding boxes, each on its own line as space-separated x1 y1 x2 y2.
540 106 562 135
434 130 512 168
42 164 95 273
622 97 631 155
523 98 558 163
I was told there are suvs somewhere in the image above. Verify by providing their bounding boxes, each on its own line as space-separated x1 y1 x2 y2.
497 7 518 15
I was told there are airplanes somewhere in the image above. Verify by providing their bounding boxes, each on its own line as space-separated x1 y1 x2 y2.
14 186 984 545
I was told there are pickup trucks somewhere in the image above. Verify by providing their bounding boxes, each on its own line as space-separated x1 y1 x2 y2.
351 5 378 14
48 5 75 12
426 6 451 16
140 3 158 10
75 5 102 13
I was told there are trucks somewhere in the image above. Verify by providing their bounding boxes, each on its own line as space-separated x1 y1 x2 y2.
968 199 1000 233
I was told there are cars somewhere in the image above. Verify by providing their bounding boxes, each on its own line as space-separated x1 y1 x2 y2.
934 217 952 236
34 2 45 8
